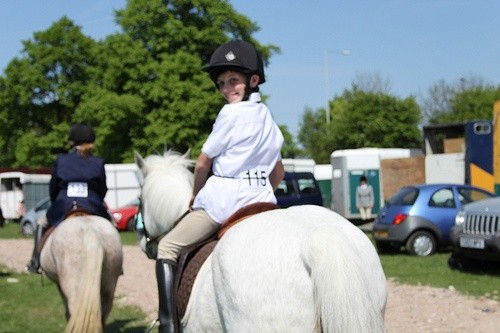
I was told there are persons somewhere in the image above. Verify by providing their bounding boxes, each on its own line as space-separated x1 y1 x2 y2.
355 175 375 224
18 200 25 221
155 39 286 333
24 120 125 276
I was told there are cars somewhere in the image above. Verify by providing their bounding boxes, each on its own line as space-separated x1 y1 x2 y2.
109 199 141 231
372 182 496 257
450 198 500 264
20 196 52 236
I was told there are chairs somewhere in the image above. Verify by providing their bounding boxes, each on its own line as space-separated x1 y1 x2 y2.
303 188 314 193
445 199 455 207
276 189 284 195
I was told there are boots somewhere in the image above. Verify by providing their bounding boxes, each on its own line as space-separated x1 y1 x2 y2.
155 258 183 333
29 225 46 271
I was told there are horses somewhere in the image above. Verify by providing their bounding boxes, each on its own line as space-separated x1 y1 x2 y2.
36 206 126 333
131 150 389 333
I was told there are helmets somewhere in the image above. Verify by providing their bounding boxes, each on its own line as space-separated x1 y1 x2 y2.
201 40 265 84
68 122 96 144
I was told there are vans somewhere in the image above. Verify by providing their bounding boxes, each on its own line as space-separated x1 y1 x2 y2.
275 171 324 207
